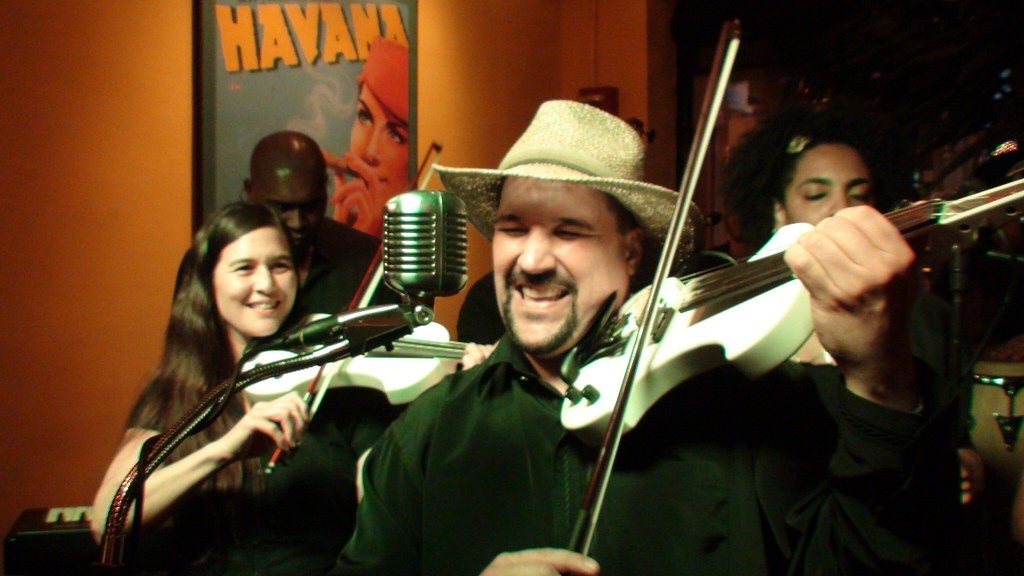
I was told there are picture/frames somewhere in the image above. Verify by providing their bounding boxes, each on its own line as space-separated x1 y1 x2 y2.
189 0 420 319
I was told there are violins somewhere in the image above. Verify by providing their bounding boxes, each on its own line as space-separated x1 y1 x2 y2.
559 175 1023 447
239 303 496 407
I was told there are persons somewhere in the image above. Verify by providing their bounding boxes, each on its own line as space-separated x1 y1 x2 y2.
243 130 409 337
321 36 410 240
91 202 499 576
328 100 941 576
722 108 985 576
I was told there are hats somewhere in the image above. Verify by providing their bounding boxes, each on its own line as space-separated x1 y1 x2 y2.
431 100 706 275
356 37 409 123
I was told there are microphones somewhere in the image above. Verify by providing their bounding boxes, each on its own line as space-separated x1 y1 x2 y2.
272 190 469 353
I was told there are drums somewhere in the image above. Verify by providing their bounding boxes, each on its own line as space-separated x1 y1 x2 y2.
967 359 1023 473
